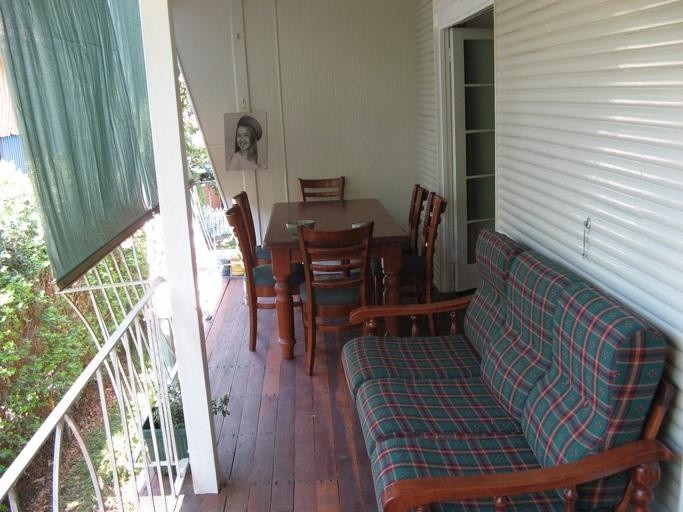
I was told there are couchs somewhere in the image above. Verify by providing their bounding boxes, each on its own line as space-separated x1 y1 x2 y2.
339 228 682 511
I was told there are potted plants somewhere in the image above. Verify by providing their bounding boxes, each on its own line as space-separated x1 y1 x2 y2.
141 376 230 478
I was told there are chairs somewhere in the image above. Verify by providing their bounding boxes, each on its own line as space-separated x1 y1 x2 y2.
296 175 345 203
372 193 447 340
223 203 312 352
295 221 377 376
230 190 306 268
372 184 426 265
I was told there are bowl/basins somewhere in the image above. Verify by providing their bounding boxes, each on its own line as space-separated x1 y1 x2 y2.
285 219 314 240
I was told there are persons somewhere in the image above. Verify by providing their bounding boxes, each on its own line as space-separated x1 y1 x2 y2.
229 115 263 170
